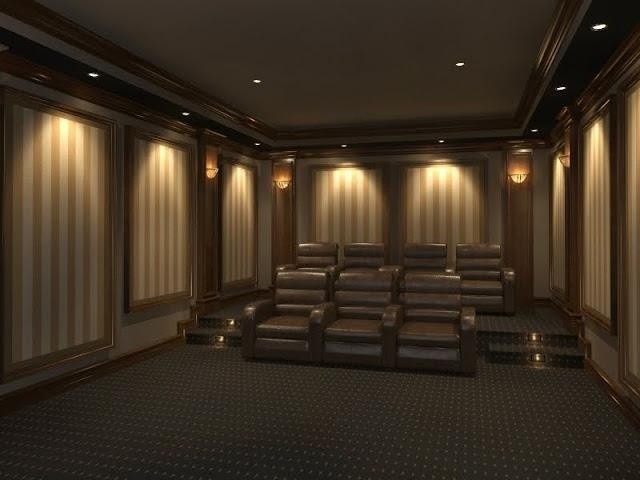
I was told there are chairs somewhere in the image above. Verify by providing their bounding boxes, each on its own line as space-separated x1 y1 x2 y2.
241 241 520 379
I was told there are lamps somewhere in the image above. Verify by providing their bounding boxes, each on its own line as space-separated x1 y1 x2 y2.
274 179 291 190
557 154 569 169
206 167 219 180
509 173 528 184
